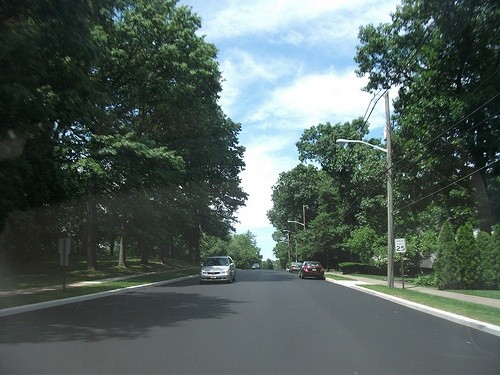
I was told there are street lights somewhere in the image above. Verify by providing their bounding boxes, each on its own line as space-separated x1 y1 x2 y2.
287 221 305 229
279 241 290 262
283 230 297 262
336 139 394 288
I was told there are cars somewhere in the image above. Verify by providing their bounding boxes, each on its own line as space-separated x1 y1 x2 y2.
252 263 260 269
289 262 303 273
299 261 324 279
200 255 236 283
